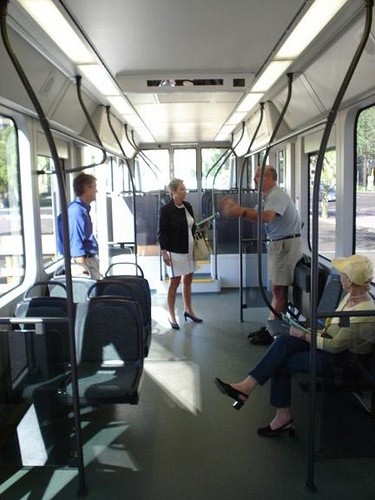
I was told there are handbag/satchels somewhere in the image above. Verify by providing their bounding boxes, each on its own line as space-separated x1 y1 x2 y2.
193 230 210 260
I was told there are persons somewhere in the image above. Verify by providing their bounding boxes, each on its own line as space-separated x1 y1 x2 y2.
223 164 303 345
57 173 100 281
158 179 203 330
214 255 375 437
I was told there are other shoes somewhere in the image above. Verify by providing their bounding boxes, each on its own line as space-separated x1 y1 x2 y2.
247 327 266 339
249 330 274 346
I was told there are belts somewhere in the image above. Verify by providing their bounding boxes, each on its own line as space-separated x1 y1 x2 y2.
267 234 302 241
82 254 96 258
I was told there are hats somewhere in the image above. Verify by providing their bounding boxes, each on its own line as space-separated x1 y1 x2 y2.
331 255 374 286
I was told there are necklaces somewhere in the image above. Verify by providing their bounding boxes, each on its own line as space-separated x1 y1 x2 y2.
348 294 369 298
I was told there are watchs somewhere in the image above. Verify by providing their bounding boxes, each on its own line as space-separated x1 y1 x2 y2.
301 332 307 341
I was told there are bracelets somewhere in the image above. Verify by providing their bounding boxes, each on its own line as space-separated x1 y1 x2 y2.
242 210 247 219
161 250 167 254
83 272 88 274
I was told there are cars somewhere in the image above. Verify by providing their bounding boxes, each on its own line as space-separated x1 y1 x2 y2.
327 189 336 202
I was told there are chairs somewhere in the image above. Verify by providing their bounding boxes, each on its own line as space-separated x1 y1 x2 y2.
265 254 375 454
119 188 265 243
0 263 152 426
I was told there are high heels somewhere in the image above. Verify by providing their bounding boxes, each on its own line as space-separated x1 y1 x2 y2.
257 418 295 438
168 318 179 329
214 377 249 411
184 312 202 322
